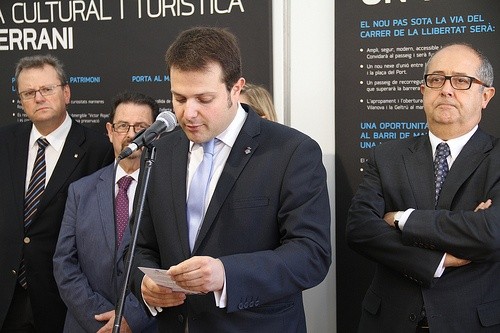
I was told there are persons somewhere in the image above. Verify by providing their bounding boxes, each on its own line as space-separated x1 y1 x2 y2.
0 55 115 333
238 82 278 123
53 90 161 333
346 41 500 333
123 27 332 333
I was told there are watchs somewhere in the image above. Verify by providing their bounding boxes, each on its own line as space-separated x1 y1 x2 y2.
394 210 406 233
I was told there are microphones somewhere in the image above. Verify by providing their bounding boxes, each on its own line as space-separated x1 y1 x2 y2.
118 111 177 157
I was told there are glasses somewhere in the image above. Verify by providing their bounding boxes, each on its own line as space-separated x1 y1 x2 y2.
423 73 491 91
111 122 151 134
19 83 64 99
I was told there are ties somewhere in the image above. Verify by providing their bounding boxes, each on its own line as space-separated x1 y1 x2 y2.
432 142 451 211
185 137 222 251
113 175 134 249
16 138 51 291
